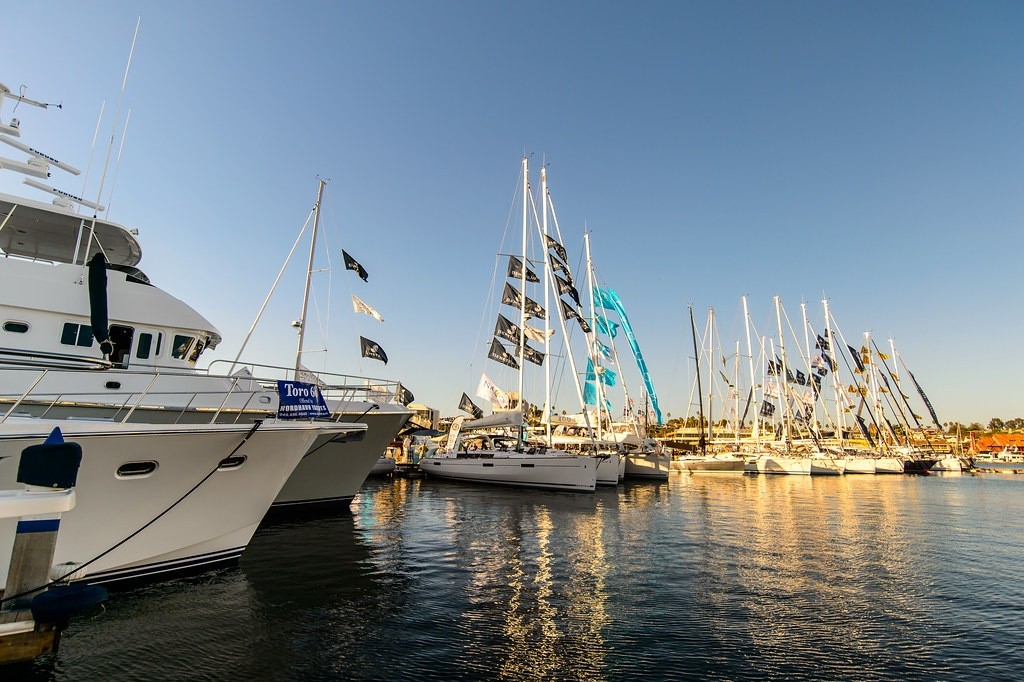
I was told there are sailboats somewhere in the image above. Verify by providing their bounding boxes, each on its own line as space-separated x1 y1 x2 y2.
670 289 980 475
230 174 417 527
419 148 670 492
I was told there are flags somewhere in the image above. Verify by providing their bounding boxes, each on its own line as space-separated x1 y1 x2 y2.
352 294 384 322
756 328 924 422
476 374 509 409
361 336 388 364
458 393 483 418
487 235 618 409
399 384 414 405
339 249 367 282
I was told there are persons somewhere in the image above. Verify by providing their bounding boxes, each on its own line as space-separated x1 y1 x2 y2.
402 436 411 460
418 443 429 460
698 434 706 455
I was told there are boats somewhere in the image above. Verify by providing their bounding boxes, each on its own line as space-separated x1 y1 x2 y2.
0 73 371 606
368 456 395 477
